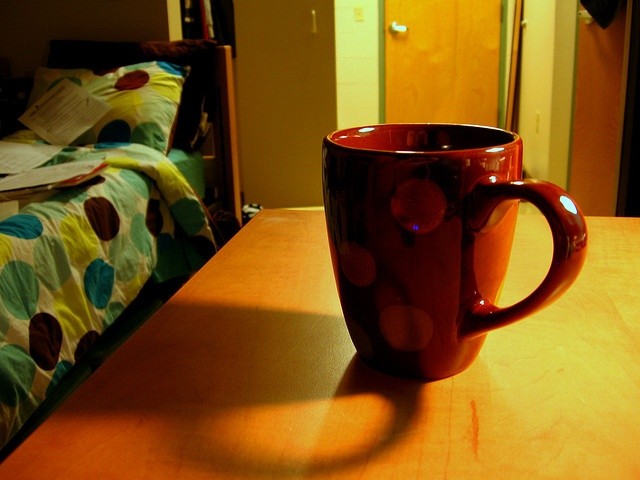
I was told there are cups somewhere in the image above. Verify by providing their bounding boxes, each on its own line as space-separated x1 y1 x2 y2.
322 124 589 383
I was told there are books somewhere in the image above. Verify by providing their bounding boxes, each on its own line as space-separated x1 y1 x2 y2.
0 164 111 199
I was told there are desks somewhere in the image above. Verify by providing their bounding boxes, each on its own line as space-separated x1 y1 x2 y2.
0 206 640 476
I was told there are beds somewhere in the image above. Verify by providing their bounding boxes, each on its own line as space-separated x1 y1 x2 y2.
0 39 241 451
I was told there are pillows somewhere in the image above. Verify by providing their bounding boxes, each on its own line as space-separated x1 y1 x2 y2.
25 59 184 155
45 40 217 150
